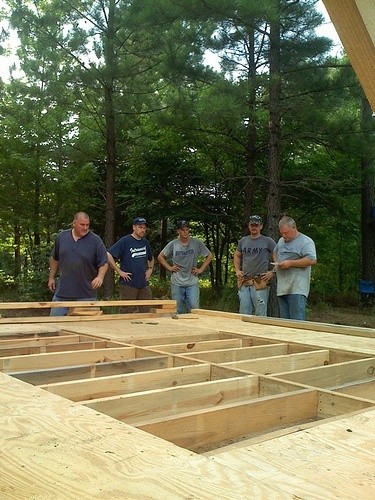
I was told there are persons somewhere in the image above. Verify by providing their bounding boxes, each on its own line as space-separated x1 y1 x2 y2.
158 220 213 314
272 216 317 320
107 217 154 314
234 215 277 317
48 212 109 316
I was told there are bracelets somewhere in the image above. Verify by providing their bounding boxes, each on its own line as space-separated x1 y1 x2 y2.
148 267 153 270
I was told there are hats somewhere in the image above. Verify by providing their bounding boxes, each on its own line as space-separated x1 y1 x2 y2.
249 215 262 224
177 220 188 229
133 217 146 225
